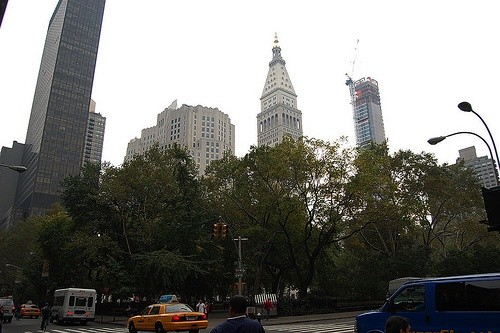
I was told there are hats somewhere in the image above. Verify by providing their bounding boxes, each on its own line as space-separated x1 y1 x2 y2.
223 295 250 307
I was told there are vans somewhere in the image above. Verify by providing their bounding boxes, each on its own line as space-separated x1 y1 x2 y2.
353 272 500 333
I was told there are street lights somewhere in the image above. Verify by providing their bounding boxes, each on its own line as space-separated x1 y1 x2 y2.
426 101 500 184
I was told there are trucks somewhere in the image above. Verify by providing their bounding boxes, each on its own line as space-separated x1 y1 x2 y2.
50 287 98 325
388 277 417 292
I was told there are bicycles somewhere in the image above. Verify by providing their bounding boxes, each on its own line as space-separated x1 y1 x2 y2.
42 320 48 331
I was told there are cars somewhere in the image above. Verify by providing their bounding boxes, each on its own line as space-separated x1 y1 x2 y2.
0 299 14 323
127 303 209 333
19 303 41 319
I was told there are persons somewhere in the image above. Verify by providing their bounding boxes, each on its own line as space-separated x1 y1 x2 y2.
202 301 209 318
125 304 139 318
207 295 266 333
263 297 274 320
195 299 205 313
0 306 5 332
15 303 22 320
40 303 51 329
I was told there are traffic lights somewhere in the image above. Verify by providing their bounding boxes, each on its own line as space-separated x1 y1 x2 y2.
213 223 218 240
481 185 500 235
221 224 227 240
